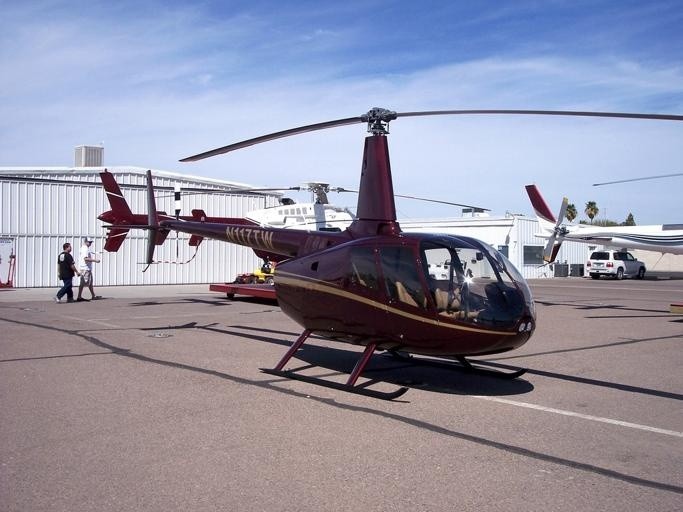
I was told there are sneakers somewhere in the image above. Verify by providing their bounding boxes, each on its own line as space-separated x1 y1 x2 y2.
66 299 77 303
91 296 102 300
76 297 89 302
52 294 61 303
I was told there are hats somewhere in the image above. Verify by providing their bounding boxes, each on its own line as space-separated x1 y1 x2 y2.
83 236 94 243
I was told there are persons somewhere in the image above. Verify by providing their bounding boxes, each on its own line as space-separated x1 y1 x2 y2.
78 235 102 301
53 242 80 304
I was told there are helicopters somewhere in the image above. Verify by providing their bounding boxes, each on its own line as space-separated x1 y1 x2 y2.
154 182 491 273
94 107 681 399
524 173 683 269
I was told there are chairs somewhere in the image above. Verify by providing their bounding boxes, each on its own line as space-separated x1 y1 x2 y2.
429 277 483 320
390 270 456 320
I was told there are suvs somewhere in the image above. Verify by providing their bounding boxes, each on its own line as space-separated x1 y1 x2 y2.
584 249 646 280
